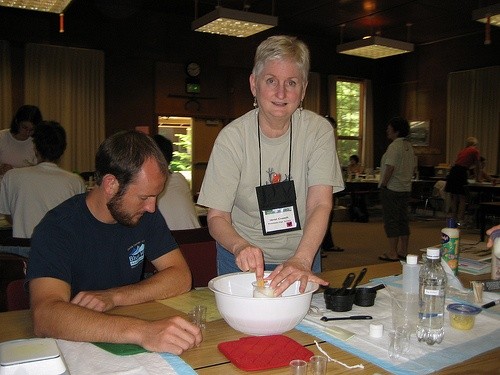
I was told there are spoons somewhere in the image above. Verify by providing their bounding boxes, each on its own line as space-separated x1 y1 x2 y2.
321 316 373 321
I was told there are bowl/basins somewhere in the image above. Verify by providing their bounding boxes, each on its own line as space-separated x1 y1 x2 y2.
209 270 320 336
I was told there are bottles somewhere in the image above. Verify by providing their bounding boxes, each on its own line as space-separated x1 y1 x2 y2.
401 254 418 292
441 217 460 276
416 247 448 345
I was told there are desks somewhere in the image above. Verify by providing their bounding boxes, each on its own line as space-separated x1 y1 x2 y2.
343 177 425 214
464 179 500 229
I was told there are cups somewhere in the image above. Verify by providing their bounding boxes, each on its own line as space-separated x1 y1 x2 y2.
388 326 410 361
391 291 417 334
309 356 326 375
188 304 206 337
324 288 354 312
354 288 376 307
290 360 307 375
252 279 276 299
446 304 479 330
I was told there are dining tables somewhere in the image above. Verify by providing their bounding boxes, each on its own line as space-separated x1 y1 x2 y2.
0 254 500 375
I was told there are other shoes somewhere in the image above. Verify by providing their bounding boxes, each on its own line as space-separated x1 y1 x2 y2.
323 246 343 252
457 223 468 229
321 252 328 258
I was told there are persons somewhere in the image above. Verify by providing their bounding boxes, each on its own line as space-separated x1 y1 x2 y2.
347 154 366 176
0 121 88 246
469 158 492 183
197 36 344 300
444 140 483 230
377 116 418 262
321 114 343 260
0 105 45 174
27 127 202 355
150 131 201 232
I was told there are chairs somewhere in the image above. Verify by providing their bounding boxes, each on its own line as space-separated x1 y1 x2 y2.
479 202 500 241
0 251 29 313
180 241 217 288
7 278 29 311
425 181 446 215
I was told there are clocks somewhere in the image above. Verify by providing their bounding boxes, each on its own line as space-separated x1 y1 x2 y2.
186 61 201 78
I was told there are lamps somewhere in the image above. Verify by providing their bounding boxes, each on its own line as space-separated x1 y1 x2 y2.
191 7 279 40
0 1 71 13
472 3 500 31
337 36 415 60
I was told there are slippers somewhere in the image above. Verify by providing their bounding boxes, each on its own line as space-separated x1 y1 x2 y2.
379 254 399 261
397 252 407 261
